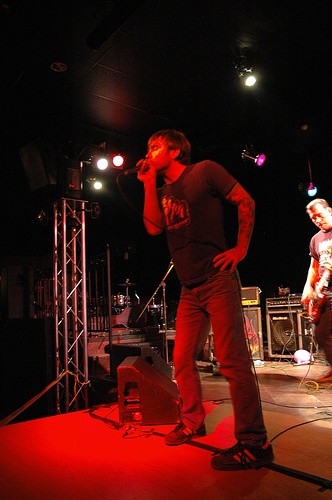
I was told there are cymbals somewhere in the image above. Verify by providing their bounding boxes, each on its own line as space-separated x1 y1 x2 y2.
119 282 138 286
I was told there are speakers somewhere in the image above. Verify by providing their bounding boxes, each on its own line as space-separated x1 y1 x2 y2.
266 308 313 358
209 307 264 361
110 343 181 425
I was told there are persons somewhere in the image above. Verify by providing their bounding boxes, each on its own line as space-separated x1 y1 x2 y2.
302 199 332 383
136 131 274 469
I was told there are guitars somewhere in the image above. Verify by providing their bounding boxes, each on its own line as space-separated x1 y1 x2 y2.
298 260 332 323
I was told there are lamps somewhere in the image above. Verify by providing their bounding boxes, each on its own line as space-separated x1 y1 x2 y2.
241 148 267 166
297 182 318 198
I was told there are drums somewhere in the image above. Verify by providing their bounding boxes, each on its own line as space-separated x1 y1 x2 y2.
111 295 125 307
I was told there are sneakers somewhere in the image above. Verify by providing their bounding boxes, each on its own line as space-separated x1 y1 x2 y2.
165 420 207 446
210 436 275 471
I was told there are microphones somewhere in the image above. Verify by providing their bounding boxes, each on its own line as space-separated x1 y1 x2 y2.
122 160 149 176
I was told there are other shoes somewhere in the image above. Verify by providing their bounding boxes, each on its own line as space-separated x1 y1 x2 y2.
314 369 332 383
